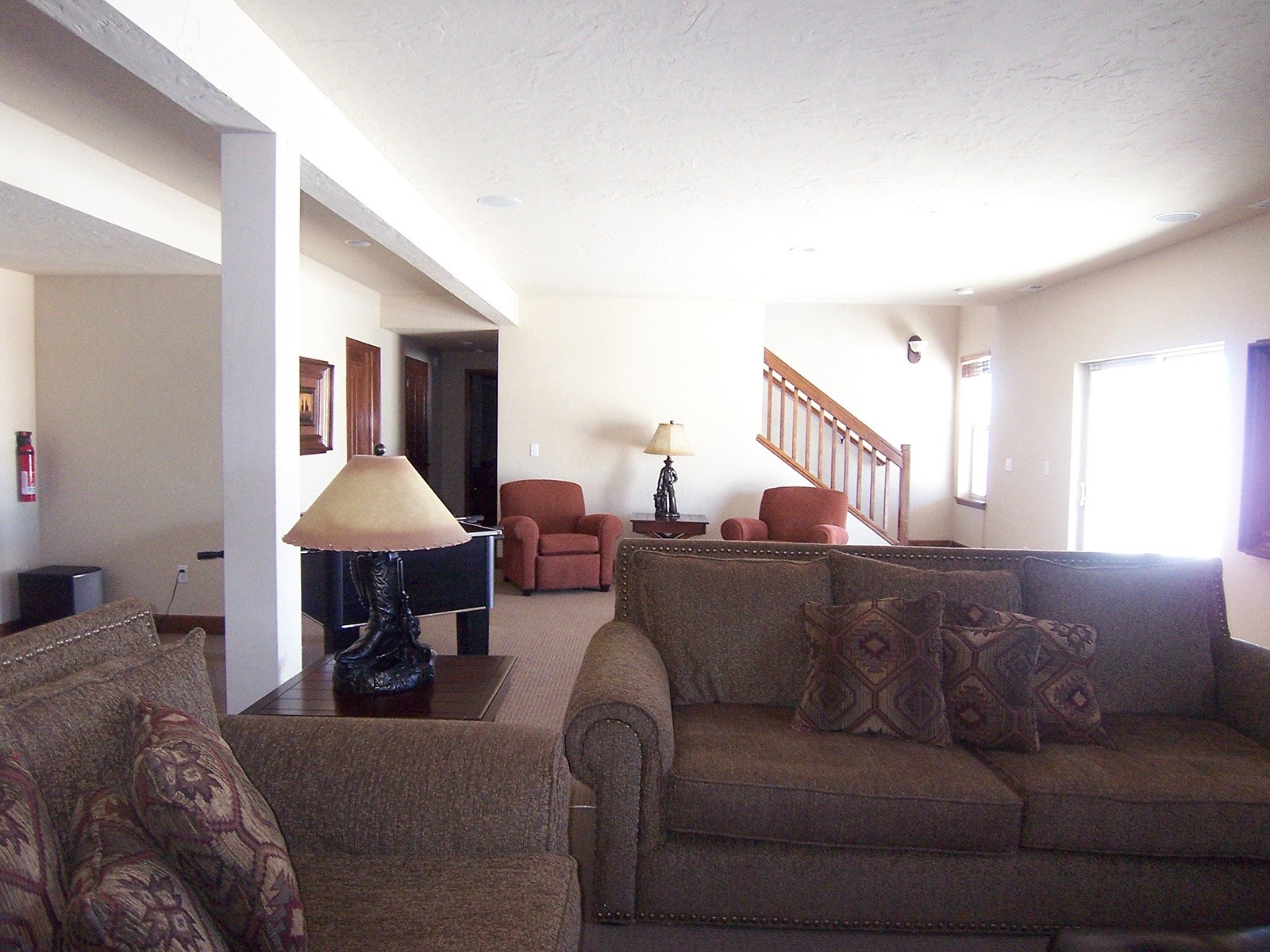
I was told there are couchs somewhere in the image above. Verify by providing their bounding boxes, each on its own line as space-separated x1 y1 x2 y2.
563 536 1270 936
498 478 622 596
720 486 851 545
2 599 583 952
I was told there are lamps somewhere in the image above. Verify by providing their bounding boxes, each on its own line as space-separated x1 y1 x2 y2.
908 334 923 363
282 451 473 693
645 422 695 518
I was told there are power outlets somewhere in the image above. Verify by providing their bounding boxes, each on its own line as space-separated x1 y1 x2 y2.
178 564 189 584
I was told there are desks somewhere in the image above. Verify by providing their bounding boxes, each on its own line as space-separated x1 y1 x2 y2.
630 512 709 539
239 650 519 722
19 564 104 630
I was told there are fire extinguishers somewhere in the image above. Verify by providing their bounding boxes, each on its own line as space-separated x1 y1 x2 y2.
18 431 36 501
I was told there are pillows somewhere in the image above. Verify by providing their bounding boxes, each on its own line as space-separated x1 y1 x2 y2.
791 588 1118 759
0 701 310 952
